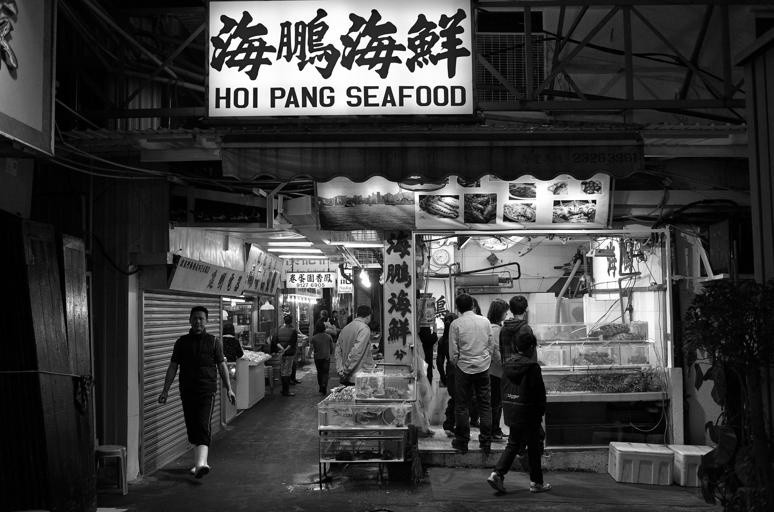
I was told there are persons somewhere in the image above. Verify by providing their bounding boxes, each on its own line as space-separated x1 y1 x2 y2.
157 306 236 479
276 315 296 396
313 309 340 396
222 322 243 362
335 306 375 386
419 295 552 494
283 316 302 385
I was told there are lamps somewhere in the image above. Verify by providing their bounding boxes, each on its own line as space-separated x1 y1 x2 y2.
258 298 274 311
285 294 317 304
356 230 372 289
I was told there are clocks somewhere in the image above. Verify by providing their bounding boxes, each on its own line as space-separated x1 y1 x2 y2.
432 249 448 265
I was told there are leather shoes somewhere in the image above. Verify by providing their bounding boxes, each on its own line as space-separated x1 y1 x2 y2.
282 379 327 397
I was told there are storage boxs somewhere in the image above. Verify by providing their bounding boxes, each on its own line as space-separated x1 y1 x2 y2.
606 440 674 486
667 444 714 486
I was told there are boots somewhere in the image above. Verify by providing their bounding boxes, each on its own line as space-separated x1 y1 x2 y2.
194 444 209 478
190 447 197 474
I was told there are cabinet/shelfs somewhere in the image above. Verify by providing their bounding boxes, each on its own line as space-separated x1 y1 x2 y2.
316 392 417 491
533 340 669 448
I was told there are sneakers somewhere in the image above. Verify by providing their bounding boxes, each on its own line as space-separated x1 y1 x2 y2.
444 420 508 451
486 471 505 493
529 481 552 492
